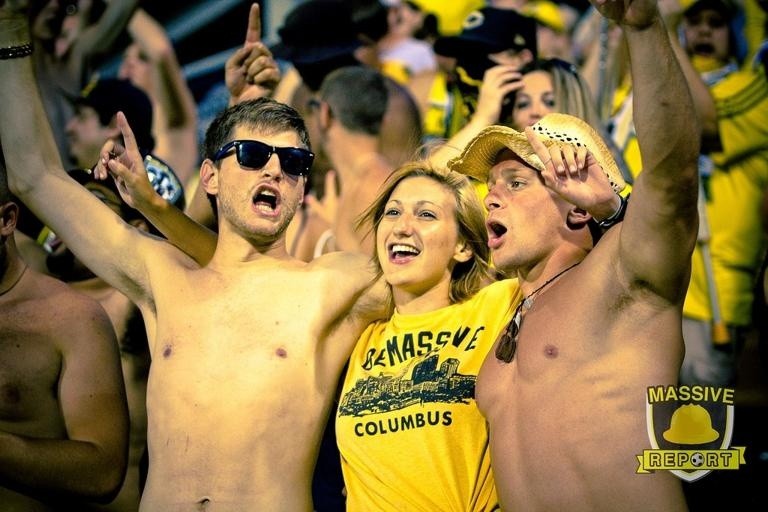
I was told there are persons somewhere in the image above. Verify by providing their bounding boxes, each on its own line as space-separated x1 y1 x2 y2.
0 1 768 511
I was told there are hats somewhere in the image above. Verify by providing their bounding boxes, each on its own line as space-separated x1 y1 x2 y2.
433 7 537 58
64 164 118 194
448 113 628 196
59 74 152 126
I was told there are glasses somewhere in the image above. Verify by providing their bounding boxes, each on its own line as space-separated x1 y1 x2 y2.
214 139 316 175
